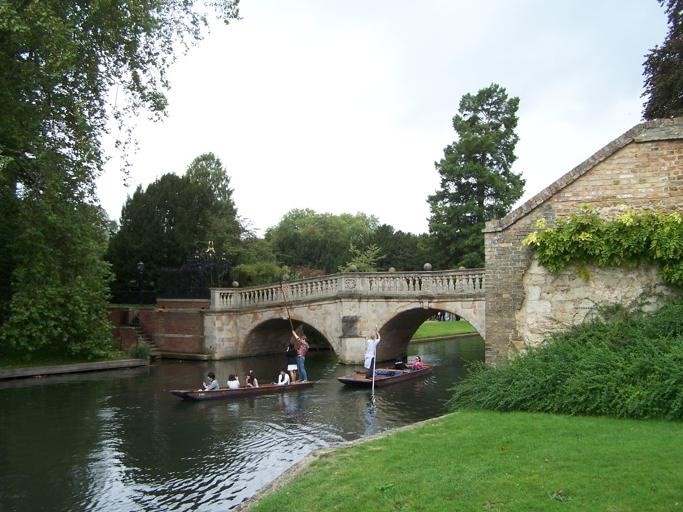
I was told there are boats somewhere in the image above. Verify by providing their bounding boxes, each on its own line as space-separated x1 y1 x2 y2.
170 381 314 400
337 362 433 386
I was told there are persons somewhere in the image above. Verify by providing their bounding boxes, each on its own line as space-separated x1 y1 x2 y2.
393 357 407 370
198 372 220 391
292 330 309 383
363 332 381 379
412 356 423 370
278 368 291 386
226 374 241 389
246 372 259 388
429 312 461 322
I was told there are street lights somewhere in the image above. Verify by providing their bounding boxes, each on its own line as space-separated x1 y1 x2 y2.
193 240 227 287
137 260 145 304
